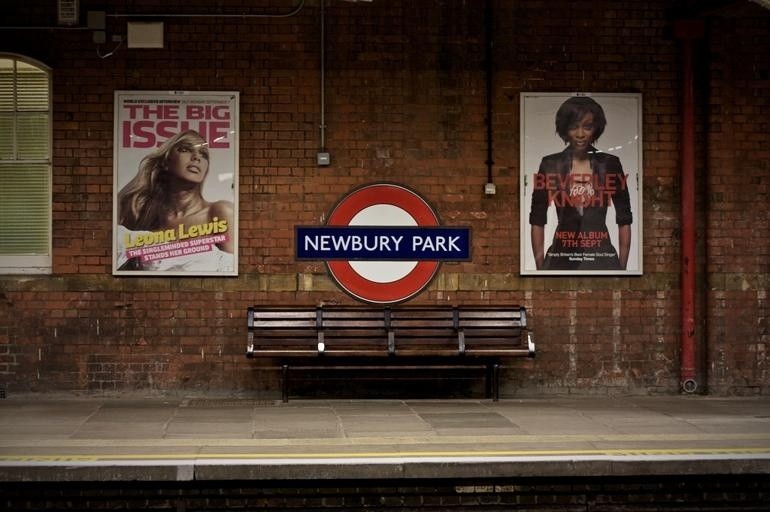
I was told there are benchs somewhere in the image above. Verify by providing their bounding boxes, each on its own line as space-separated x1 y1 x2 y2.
247 304 537 401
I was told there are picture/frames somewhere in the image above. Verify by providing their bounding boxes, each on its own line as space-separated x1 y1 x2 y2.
519 92 643 276
115 91 240 277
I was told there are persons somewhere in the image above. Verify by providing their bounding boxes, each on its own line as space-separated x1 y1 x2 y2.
528 94 634 272
115 131 234 274
